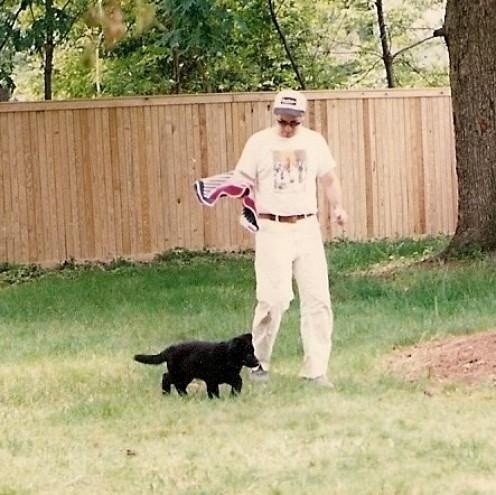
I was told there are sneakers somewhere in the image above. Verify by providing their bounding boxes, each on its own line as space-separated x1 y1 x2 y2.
249 368 270 384
301 376 332 389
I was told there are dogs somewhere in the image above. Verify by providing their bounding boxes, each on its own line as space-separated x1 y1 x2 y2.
132 332 259 400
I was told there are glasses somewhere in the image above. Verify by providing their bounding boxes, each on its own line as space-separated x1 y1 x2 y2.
277 119 301 126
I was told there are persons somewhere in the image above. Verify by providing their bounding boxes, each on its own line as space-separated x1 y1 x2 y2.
193 88 351 391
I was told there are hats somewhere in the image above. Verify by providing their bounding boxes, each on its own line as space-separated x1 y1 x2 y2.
272 90 307 115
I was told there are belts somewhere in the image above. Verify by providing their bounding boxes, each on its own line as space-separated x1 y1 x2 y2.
255 213 313 224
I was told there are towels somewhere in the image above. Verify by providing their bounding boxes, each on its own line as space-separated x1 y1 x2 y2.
193 172 260 234
